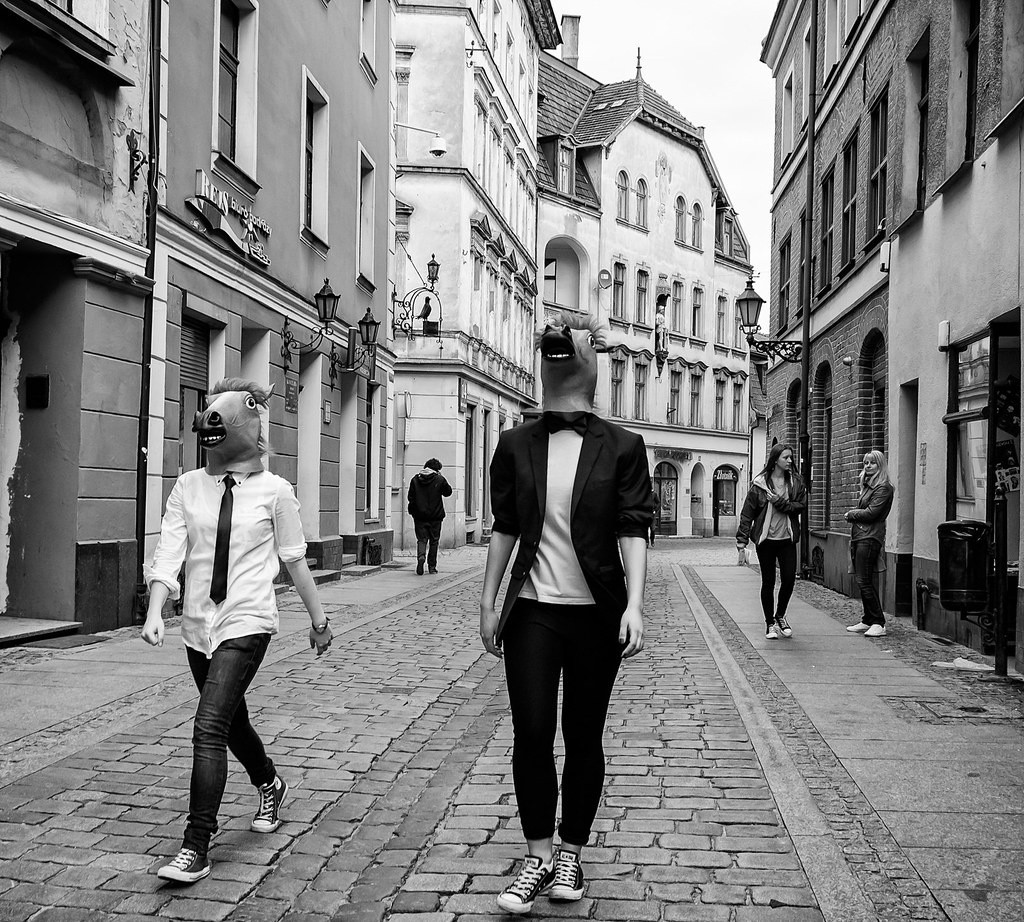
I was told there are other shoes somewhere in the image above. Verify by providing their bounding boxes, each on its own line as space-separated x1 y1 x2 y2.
429 568 439 575
416 556 426 575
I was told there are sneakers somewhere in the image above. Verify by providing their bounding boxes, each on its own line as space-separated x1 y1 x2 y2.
846 622 870 632
765 621 778 639
548 848 584 900
157 848 211 882
497 854 556 913
774 615 793 638
251 775 289 833
863 624 887 636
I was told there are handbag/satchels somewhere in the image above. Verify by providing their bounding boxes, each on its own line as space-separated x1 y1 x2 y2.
847 536 887 574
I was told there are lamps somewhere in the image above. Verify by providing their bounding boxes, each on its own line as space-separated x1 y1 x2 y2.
391 255 439 340
740 275 803 363
281 278 341 374
330 307 380 389
394 124 447 158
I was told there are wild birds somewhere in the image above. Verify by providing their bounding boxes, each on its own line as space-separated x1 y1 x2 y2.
415 296 431 321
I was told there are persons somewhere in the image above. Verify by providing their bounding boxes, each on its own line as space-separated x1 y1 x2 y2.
140 378 332 885
656 305 666 351
735 443 807 640
647 480 660 549
477 311 655 914
408 458 452 575
843 450 895 636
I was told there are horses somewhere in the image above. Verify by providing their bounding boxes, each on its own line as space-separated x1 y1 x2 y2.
190 379 276 476
534 313 607 412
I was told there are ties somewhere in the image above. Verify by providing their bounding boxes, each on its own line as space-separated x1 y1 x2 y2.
209 475 237 606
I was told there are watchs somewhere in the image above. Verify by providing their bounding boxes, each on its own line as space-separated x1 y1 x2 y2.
312 617 330 634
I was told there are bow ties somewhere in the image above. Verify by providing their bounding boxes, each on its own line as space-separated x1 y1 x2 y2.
543 410 589 438
